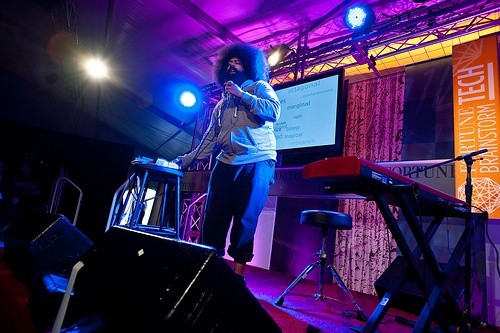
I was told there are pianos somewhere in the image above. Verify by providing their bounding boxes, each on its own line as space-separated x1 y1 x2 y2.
302 156 471 218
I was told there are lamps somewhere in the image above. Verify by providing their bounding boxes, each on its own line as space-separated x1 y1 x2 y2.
343 3 377 33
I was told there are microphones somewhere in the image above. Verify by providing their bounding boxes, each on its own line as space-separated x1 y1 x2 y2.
226 79 234 107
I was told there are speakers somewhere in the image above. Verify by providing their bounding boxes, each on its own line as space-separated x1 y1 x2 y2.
374 255 483 320
68 226 285 333
3 210 94 270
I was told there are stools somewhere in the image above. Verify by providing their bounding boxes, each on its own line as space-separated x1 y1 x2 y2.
272 210 369 323
117 163 185 239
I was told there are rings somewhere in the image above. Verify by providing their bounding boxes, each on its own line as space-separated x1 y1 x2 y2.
228 81 231 86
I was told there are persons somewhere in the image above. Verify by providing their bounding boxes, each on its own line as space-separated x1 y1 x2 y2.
92 207 110 242
174 42 281 280
